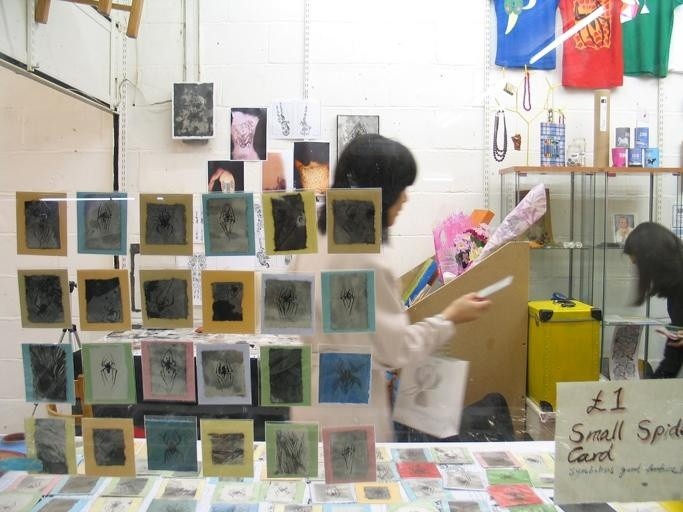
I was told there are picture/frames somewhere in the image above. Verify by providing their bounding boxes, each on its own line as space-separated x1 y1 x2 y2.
171 81 217 140
612 213 637 243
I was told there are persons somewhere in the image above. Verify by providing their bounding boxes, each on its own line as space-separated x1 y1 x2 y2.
314 131 496 450
208 166 236 194
622 219 682 379
614 216 634 245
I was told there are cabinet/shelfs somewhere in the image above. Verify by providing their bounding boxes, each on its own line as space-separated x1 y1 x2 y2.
498 164 683 379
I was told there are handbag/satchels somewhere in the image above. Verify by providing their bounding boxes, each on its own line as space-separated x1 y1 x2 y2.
392 355 469 439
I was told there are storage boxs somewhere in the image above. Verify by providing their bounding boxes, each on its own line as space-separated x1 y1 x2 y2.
524 298 602 440
